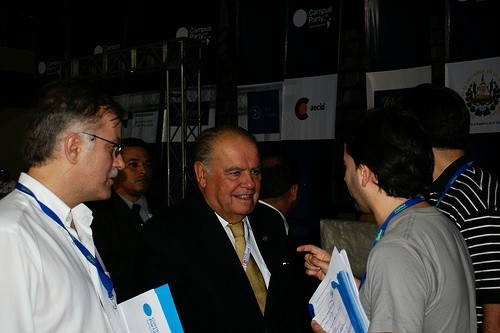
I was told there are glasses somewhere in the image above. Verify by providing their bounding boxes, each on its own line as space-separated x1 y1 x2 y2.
81 132 122 157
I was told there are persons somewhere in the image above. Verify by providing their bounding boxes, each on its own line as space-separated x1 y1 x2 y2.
296 104 478 333
396 84 500 333
84 125 312 333
0 89 126 333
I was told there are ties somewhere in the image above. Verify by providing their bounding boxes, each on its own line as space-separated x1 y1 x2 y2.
131 203 145 227
225 221 268 316
